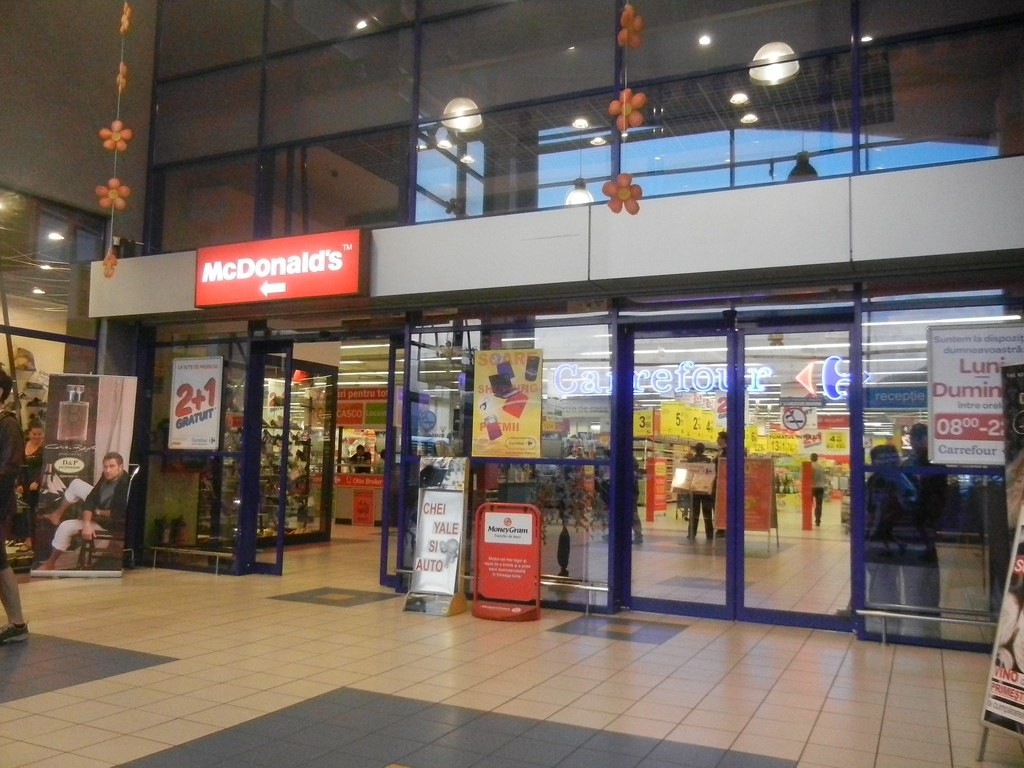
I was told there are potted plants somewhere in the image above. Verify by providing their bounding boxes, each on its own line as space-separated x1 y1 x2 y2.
171 515 190 546
153 513 171 546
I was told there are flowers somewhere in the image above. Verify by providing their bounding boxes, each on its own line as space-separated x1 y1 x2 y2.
119 3 132 35
116 61 129 96
602 173 642 216
609 89 647 131
95 177 131 210
616 4 644 48
98 120 134 152
101 246 119 278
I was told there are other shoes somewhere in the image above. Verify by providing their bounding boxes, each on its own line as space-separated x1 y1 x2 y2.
198 395 315 538
3 381 48 422
16 543 32 553
632 537 643 544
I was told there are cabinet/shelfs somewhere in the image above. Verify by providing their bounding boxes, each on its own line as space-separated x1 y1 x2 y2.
197 353 339 549
635 437 719 503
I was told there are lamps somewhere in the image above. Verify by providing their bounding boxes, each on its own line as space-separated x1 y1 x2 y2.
749 42 800 86
441 97 484 134
437 140 453 149
786 150 818 182
461 155 475 164
566 129 594 205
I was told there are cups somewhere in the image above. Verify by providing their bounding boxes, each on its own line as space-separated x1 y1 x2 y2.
485 416 501 441
525 356 539 382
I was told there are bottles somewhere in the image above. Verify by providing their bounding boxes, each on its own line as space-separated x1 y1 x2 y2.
58 385 90 443
38 464 56 501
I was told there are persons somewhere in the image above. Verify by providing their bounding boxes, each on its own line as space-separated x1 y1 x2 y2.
809 452 826 526
710 431 728 538
349 444 372 474
35 450 134 571
687 443 716 541
1 368 31 644
11 416 49 554
559 443 598 528
862 423 950 636
601 443 644 545
373 449 386 474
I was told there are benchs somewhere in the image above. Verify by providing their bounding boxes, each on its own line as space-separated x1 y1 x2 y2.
147 547 234 576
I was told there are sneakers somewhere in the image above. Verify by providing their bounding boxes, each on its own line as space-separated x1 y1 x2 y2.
0 621 29 644
